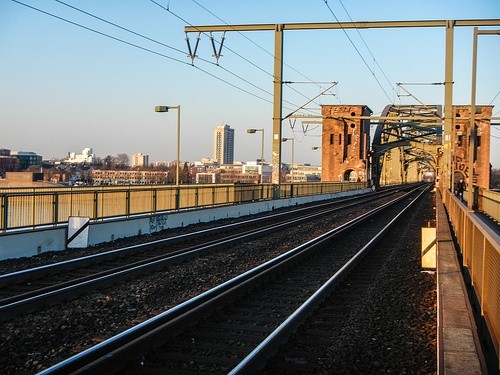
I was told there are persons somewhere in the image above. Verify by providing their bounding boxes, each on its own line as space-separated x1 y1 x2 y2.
456 178 466 198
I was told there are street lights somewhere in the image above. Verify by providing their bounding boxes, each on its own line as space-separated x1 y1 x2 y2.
279 138 295 182
155 104 181 184
247 128 264 183
